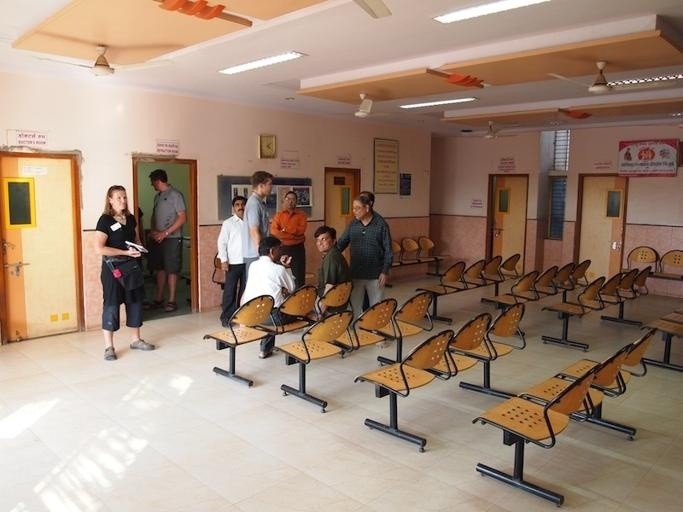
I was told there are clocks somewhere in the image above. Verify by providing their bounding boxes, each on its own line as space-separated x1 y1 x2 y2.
259 134 277 159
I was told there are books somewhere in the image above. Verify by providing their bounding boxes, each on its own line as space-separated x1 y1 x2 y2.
125 240 148 253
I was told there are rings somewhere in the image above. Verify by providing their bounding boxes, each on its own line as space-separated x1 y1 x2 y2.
158 237 160 241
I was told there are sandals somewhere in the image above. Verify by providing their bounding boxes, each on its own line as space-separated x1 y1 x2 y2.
144 300 176 312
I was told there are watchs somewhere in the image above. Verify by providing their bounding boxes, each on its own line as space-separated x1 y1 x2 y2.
163 230 170 236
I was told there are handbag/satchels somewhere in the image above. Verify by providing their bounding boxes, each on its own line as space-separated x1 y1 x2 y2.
113 259 145 292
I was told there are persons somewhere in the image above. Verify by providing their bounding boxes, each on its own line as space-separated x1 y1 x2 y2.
138 208 151 310
361 191 389 310
314 226 351 318
217 195 246 328
240 170 274 288
148 169 187 312
270 191 308 297
335 195 393 333
239 236 299 358
95 185 155 360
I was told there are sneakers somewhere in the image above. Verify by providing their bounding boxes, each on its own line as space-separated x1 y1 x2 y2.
105 348 116 361
131 339 154 351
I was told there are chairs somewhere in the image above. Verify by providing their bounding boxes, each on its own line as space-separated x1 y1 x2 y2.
381 236 447 288
541 264 654 352
472 325 665 505
204 278 356 387
353 300 528 453
618 245 682 295
639 309 683 372
280 290 435 414
414 253 523 326
480 257 593 336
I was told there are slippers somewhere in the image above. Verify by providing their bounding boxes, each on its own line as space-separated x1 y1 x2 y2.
260 351 271 359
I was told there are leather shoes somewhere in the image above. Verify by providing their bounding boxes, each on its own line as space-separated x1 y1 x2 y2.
221 319 228 327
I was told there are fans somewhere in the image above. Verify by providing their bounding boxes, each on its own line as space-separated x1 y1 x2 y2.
40 45 176 78
333 95 402 119
471 120 519 140
353 1 392 20
545 61 675 98
